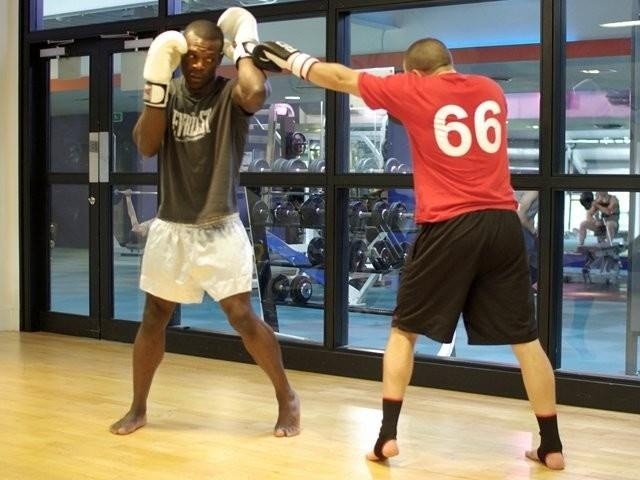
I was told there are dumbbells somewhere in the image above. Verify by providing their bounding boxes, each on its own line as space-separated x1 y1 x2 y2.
247 154 325 176
255 195 325 267
266 274 312 304
347 197 414 273
353 156 412 174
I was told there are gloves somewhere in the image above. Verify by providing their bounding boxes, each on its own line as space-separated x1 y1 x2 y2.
252 40 321 81
142 30 188 109
217 6 259 68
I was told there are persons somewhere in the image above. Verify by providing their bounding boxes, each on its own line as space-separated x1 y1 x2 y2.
251 38 565 472
514 187 541 296
106 7 304 437
575 190 620 245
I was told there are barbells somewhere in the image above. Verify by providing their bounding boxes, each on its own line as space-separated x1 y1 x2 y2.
112 185 158 205
